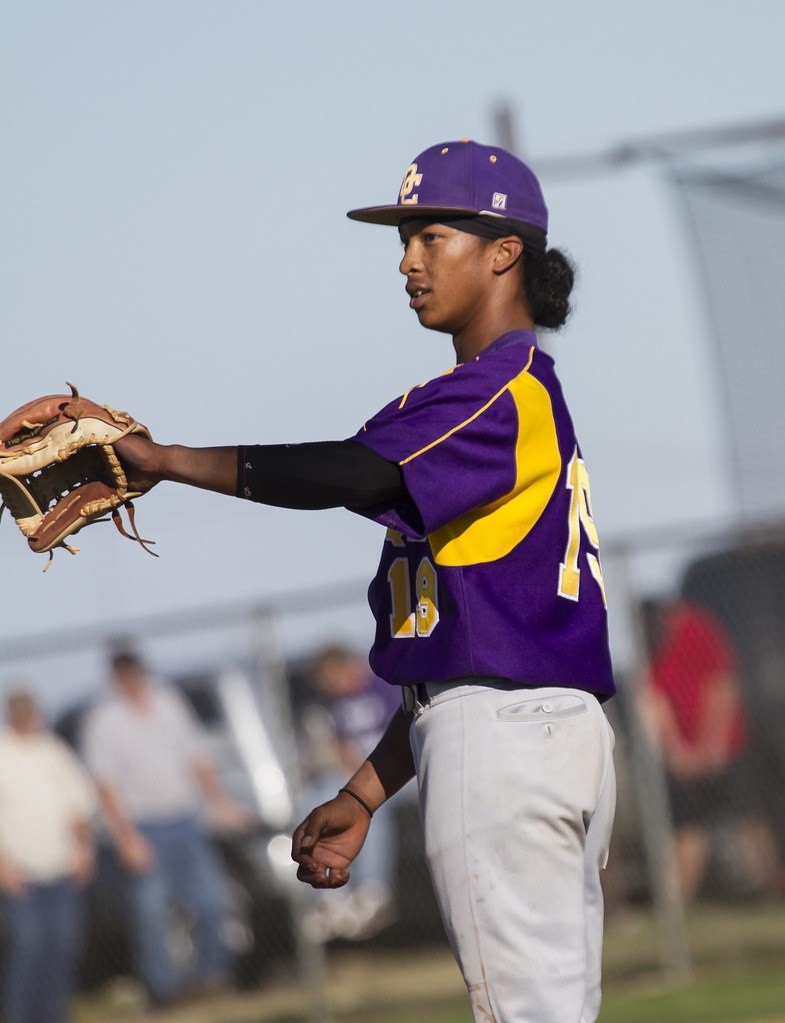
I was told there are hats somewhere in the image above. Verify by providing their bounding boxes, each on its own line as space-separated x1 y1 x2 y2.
347 138 550 233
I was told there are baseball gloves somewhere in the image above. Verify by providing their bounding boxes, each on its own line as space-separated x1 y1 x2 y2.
0 394 158 554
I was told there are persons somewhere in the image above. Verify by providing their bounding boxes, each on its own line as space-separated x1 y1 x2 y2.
219 649 402 945
631 558 773 906
0 138 615 1023
79 651 242 1007
0 694 101 1023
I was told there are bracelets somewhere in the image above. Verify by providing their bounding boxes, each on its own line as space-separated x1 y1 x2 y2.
338 788 373 819
